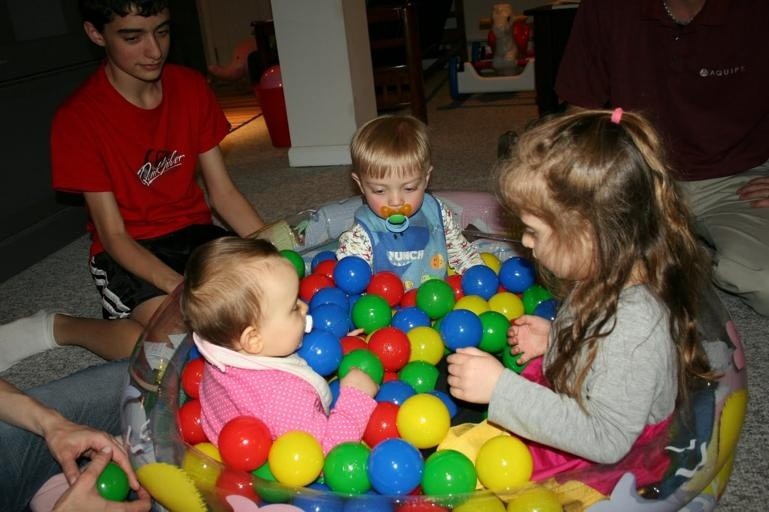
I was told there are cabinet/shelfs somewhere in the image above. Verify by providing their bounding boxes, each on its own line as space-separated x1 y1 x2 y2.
366 1 468 126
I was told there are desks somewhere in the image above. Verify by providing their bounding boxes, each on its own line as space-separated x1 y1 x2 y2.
523 2 580 118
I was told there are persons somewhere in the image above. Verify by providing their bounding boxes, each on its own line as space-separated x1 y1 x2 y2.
3 1 266 371
446 102 720 499
180 232 381 470
0 374 151 512
555 2 769 318
328 110 487 276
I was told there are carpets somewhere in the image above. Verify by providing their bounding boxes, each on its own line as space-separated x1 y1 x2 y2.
214 91 264 132
